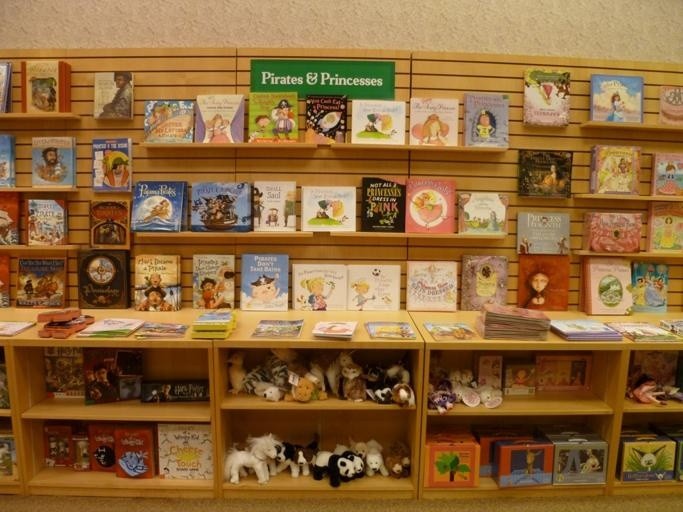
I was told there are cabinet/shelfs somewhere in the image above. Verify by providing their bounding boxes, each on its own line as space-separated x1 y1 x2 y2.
212 310 424 501
0 112 683 258
409 310 683 502
0 307 218 499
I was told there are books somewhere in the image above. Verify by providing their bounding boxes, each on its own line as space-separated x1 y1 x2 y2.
141 67 683 344
1 311 146 337
1 59 132 311
0 345 213 480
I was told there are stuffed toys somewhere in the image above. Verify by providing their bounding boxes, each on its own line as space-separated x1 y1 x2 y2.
224 348 416 406
428 361 503 411
224 347 682 489
225 433 412 489
